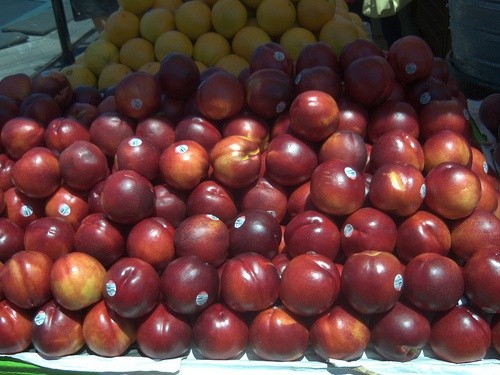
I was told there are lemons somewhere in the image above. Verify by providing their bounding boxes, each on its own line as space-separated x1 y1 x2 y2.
62 0 371 91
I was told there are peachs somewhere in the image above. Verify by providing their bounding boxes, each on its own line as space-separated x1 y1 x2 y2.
0 36 500 363
